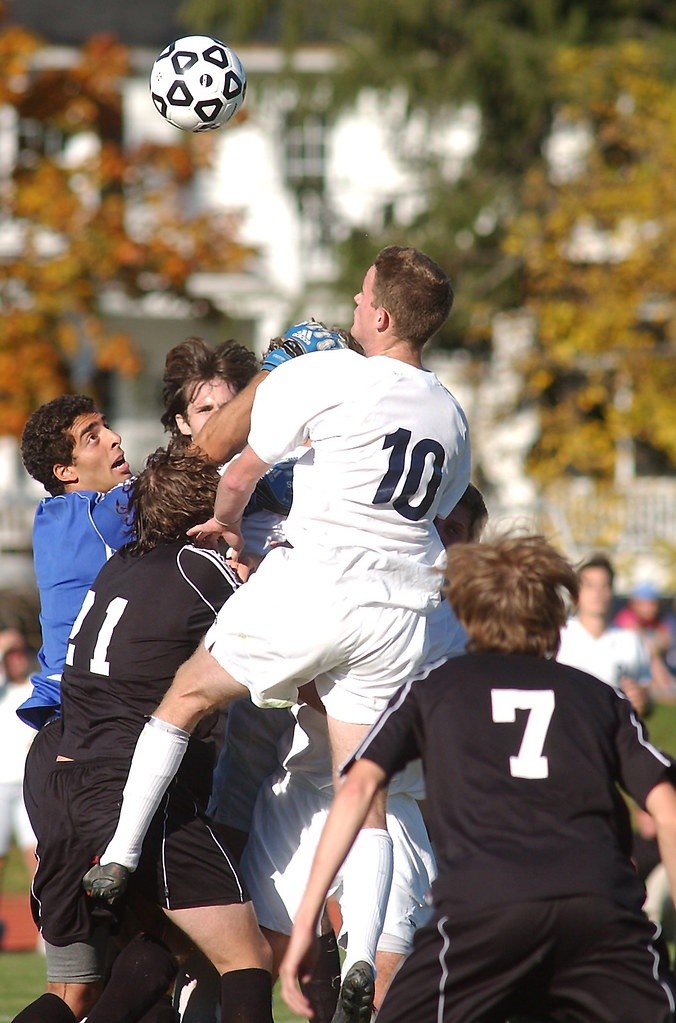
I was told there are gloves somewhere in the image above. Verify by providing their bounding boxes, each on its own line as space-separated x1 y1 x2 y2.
258 317 366 373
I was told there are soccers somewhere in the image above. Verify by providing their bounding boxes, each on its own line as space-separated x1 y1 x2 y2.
149 33 248 134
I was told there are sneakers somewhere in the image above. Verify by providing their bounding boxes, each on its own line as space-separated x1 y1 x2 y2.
330 960 378 1023
82 853 130 904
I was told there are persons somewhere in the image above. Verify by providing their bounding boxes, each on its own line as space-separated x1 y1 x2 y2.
553 551 652 715
0 245 676 1023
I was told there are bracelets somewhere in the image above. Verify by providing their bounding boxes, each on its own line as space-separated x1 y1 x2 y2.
214 515 242 526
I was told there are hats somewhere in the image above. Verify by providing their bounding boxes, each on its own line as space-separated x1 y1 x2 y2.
633 583 659 599
568 549 611 573
